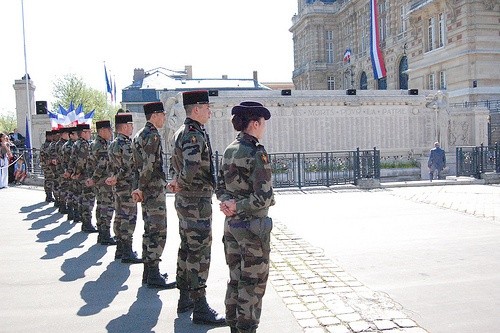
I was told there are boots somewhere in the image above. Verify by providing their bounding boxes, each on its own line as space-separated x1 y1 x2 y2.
67 204 82 222
143 273 168 282
115 239 137 258
147 280 176 289
102 230 116 245
59 201 69 214
177 290 194 313
193 297 228 326
121 240 142 263
82 215 98 233
46 192 56 202
97 230 113 242
54 197 60 207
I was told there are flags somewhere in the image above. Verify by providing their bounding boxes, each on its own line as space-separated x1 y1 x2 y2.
105 65 116 103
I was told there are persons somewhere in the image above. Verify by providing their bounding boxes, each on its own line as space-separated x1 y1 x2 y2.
167 91 226 327
86 121 116 245
428 142 447 180
131 102 176 289
39 123 98 233
105 114 143 263
216 102 275 333
0 128 26 189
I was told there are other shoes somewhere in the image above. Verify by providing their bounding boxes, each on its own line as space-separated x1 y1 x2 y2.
229 322 257 333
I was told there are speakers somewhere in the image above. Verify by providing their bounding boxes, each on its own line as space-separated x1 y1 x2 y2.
209 90 218 96
346 89 356 95
408 89 418 95
281 90 291 95
36 101 47 114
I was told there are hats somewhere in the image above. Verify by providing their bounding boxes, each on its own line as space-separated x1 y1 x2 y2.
76 124 89 131
143 102 165 115
52 130 60 135
231 100 271 120
60 128 69 134
69 127 77 132
96 120 110 129
46 131 51 135
115 113 133 124
182 90 209 105
